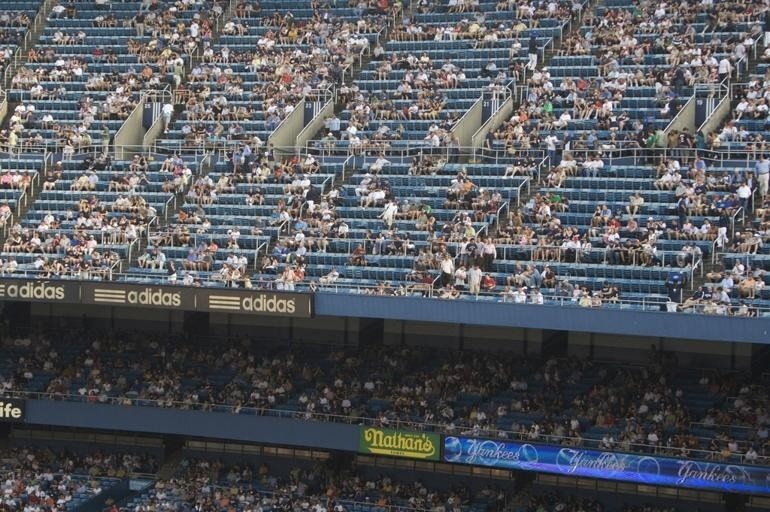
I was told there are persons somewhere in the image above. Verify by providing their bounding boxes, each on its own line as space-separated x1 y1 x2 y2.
0 1 769 510
